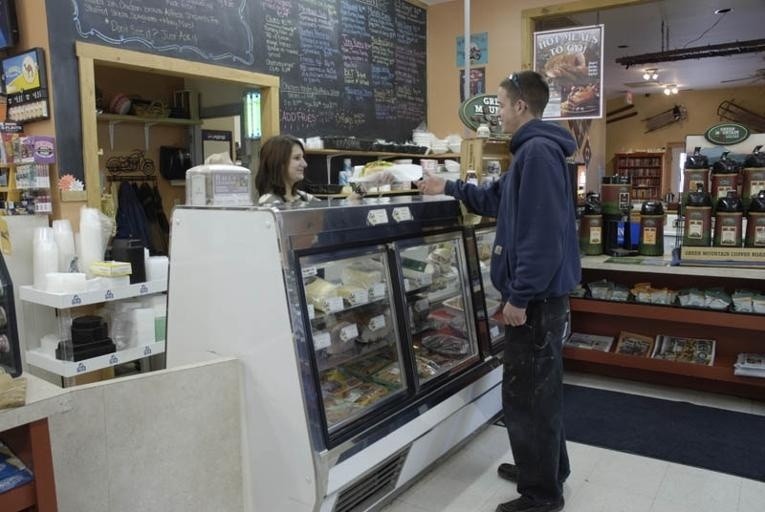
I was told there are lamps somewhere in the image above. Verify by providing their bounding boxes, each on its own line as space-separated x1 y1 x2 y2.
642 67 659 82
663 83 679 96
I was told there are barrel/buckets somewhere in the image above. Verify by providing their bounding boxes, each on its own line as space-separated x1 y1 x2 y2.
183 152 252 210
715 212 746 247
683 167 709 203
580 213 604 255
711 172 738 206
683 205 713 247
600 183 633 216
640 215 664 255
744 213 765 248
741 168 764 203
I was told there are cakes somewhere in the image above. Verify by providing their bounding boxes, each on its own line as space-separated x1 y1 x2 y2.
560 84 600 117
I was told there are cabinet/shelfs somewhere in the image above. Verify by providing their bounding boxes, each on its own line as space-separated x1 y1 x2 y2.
559 132 765 481
614 152 671 200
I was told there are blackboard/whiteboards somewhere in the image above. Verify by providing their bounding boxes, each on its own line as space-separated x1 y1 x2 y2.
46 0 427 190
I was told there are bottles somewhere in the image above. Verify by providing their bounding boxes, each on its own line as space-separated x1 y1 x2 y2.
475 124 491 138
464 171 479 187
126 233 147 285
619 157 662 199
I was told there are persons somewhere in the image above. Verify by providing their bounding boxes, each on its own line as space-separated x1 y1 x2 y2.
256 134 366 207
413 70 582 511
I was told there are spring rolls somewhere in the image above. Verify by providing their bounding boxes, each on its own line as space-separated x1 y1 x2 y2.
544 51 586 77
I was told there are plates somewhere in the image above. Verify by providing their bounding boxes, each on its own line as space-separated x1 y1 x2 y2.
412 131 464 154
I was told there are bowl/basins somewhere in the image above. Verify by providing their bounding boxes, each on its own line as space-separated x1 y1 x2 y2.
386 164 423 183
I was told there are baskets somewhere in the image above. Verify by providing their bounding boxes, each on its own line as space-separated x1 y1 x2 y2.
133 99 170 118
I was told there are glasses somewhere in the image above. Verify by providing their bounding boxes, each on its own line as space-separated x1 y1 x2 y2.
509 72 521 99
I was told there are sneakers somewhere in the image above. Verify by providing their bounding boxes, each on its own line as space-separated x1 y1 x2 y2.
496 494 564 512
499 463 520 482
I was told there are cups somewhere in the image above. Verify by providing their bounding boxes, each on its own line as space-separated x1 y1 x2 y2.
147 255 168 283
131 308 156 350
31 208 104 291
